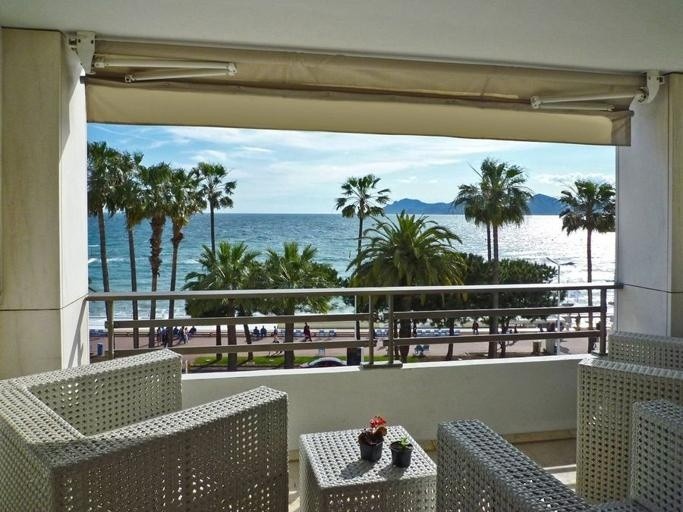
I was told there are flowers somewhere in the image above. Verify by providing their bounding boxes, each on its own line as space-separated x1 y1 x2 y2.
360 415 386 446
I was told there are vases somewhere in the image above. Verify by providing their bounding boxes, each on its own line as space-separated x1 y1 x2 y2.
357 433 385 462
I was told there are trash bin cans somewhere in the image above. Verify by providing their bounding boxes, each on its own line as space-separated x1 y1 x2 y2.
96 343 103 355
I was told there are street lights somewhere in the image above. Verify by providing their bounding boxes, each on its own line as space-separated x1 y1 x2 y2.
547 258 574 357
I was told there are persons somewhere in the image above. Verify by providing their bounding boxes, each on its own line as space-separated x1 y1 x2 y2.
510 322 564 344
575 314 601 350
472 320 479 334
157 325 196 346
253 322 312 343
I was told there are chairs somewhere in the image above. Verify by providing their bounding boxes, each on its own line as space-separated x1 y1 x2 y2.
0 348 289 512
433 329 683 512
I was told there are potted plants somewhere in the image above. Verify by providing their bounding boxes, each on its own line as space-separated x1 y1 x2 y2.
389 434 414 466
